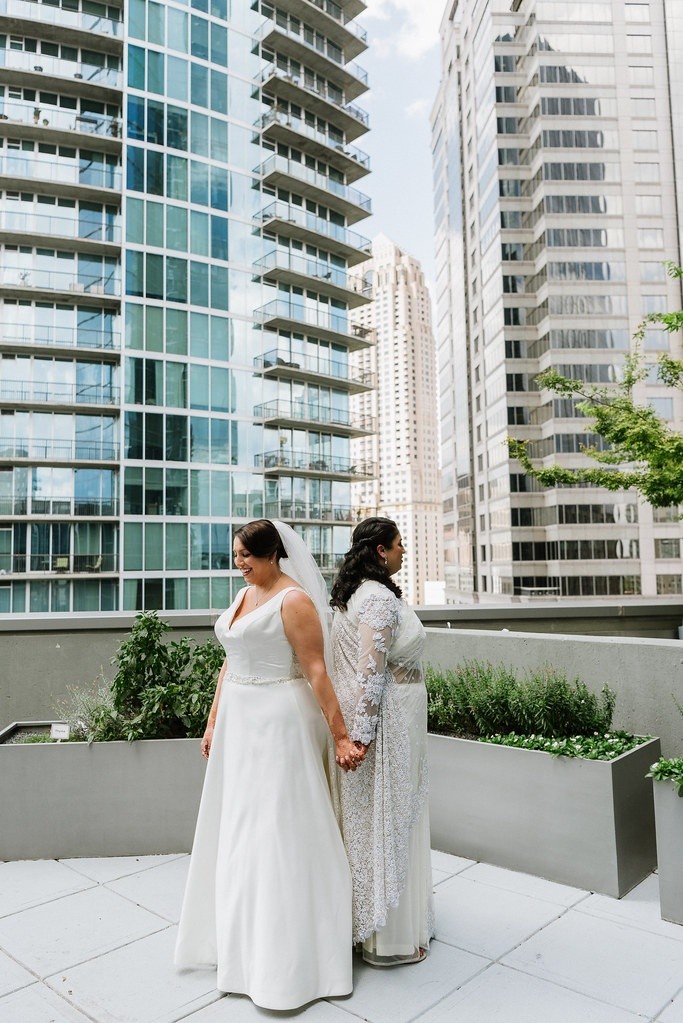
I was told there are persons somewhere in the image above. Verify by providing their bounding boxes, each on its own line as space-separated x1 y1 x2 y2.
326 517 439 968
165 519 369 1012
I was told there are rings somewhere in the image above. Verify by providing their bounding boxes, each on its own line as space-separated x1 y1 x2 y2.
350 757 356 763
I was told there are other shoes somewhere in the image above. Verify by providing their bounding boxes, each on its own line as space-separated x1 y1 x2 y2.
392 948 426 963
